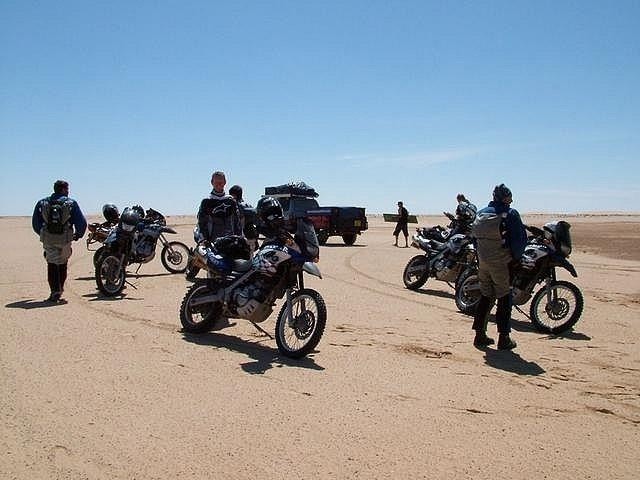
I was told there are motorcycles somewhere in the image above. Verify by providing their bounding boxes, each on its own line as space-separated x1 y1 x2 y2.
93 206 154 296
179 214 327 359
87 205 189 274
455 221 584 335
403 212 468 288
185 227 206 280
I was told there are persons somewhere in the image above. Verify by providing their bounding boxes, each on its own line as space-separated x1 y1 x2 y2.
393 202 409 248
33 180 87 306
472 183 527 350
229 185 260 259
452 194 477 234
197 172 245 242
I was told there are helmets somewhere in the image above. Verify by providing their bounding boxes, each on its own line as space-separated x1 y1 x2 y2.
103 204 119 219
260 196 284 222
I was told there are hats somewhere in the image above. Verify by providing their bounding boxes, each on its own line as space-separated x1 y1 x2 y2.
493 184 512 201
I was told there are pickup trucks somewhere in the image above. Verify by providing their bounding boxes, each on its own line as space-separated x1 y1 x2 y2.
261 193 368 246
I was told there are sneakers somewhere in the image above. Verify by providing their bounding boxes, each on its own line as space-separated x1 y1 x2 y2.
51 292 62 302
44 299 57 305
498 335 517 350
474 336 494 345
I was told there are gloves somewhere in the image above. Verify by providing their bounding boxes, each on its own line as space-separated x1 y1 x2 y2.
73 233 79 241
